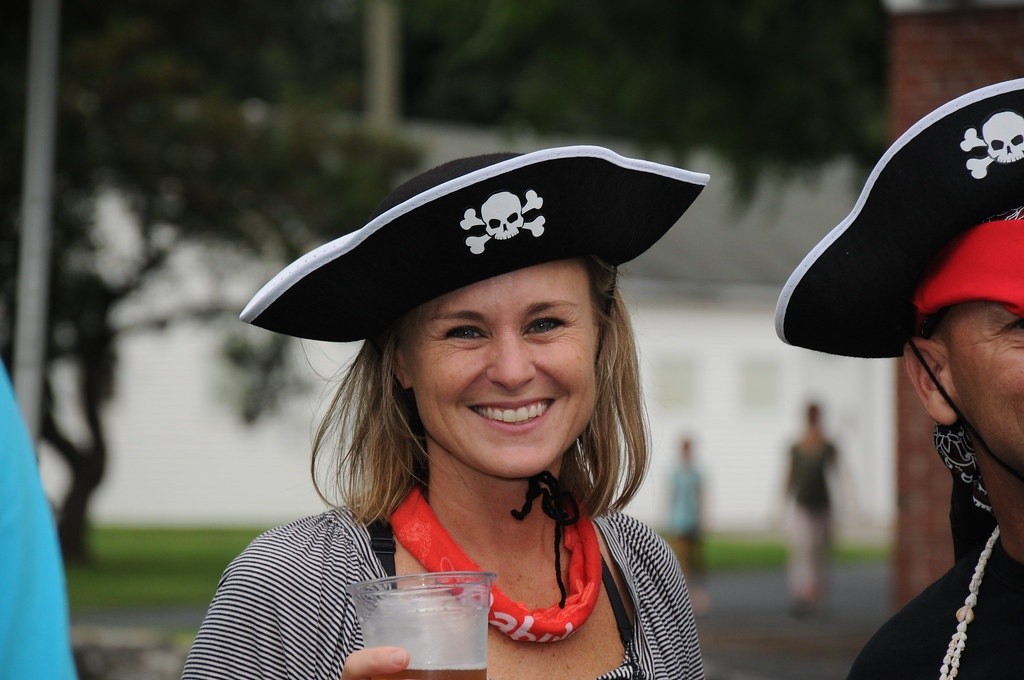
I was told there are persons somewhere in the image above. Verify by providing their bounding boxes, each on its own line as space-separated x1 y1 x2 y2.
775 77 1024 680
0 355 80 680
180 144 712 680
669 441 704 590
784 405 836 618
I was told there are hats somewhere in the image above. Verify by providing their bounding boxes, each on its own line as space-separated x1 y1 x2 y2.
774 78 1024 358
239 146 711 343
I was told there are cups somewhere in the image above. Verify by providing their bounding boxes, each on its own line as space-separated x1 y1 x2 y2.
347 572 496 680
